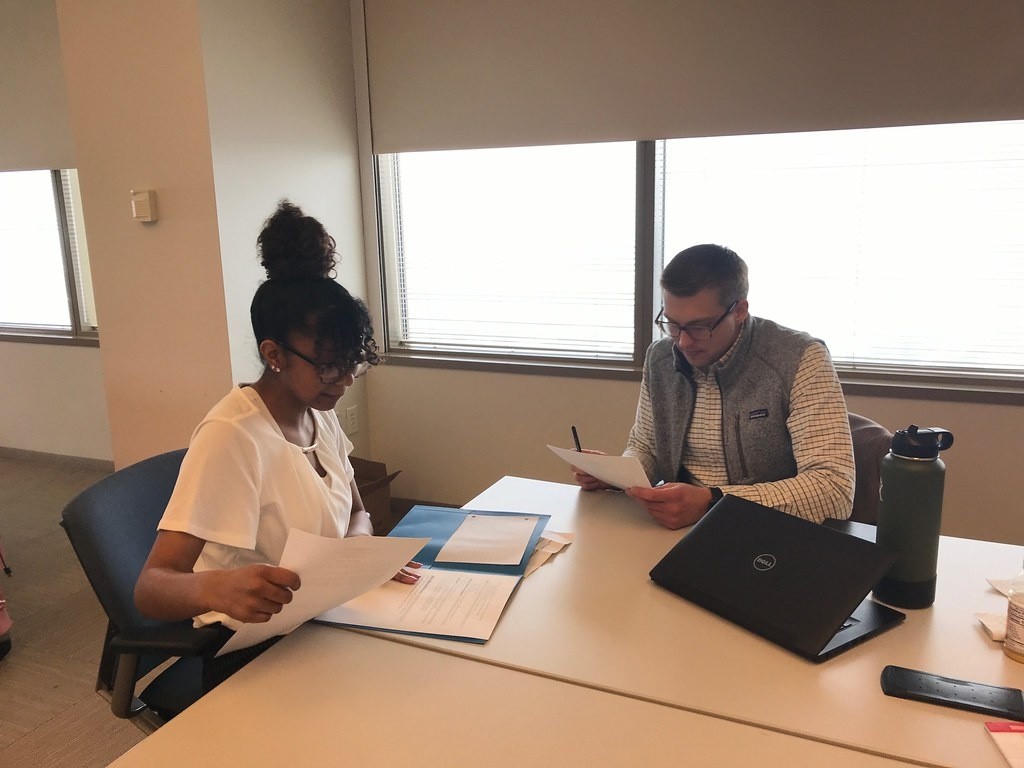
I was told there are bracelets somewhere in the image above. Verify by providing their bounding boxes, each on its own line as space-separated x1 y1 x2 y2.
708 486 723 511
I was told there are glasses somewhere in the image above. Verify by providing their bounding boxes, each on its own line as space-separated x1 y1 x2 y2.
655 301 739 340
263 335 377 384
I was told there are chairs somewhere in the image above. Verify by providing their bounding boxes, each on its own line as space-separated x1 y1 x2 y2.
60 446 220 738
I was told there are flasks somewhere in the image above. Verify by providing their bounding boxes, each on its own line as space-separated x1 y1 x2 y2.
869 423 955 611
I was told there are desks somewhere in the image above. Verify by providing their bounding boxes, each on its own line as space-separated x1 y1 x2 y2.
100 474 1024 768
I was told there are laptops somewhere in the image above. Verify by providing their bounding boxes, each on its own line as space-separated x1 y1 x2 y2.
649 493 906 662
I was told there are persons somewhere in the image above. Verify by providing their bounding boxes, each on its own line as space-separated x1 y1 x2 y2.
131 199 426 720
565 242 858 531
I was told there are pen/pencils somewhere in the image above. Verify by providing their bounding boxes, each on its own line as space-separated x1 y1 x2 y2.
572 425 582 454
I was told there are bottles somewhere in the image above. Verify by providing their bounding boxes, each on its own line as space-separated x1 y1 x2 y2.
1001 559 1024 664
